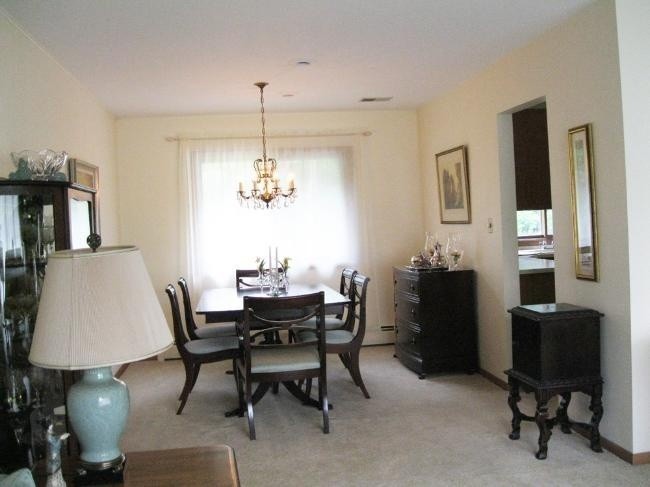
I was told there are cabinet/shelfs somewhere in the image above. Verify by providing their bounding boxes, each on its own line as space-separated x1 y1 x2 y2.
390 262 479 381
0 178 101 456
502 303 605 461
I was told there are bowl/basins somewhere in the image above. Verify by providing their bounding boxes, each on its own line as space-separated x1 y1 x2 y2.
10 148 69 179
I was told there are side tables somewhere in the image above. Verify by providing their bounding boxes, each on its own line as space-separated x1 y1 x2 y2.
29 444 241 487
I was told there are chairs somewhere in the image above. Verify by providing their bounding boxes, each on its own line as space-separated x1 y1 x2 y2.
297 268 358 369
298 274 370 407
235 290 328 441
164 284 242 418
236 269 285 288
178 277 242 392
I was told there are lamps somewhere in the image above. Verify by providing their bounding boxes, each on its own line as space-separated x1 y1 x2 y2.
237 82 298 210
28 234 175 473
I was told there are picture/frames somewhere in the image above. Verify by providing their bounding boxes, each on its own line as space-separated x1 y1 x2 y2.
435 145 471 225
567 123 600 284
